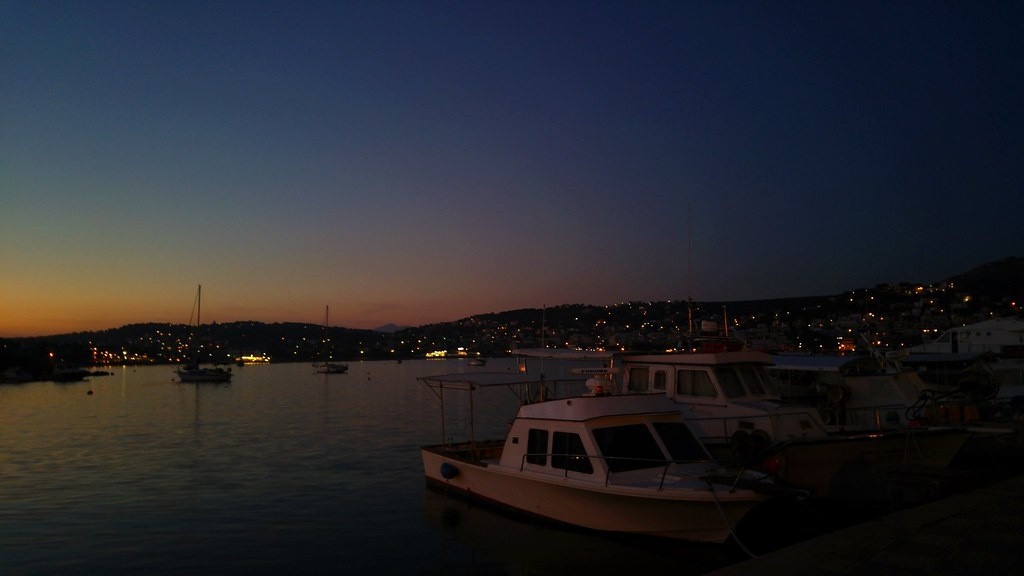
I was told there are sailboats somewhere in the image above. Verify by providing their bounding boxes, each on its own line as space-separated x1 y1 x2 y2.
311 305 349 374
172 285 233 382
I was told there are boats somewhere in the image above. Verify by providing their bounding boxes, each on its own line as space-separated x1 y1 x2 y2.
414 315 1023 544
